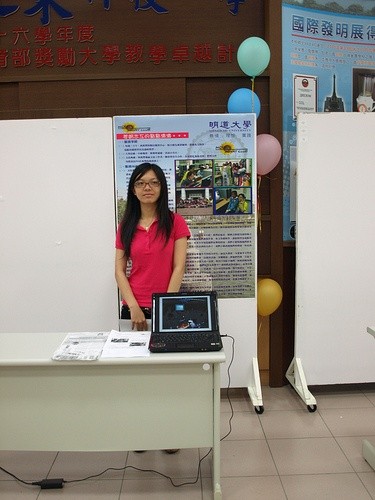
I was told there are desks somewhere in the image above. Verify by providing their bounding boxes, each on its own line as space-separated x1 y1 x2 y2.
0 332 225 500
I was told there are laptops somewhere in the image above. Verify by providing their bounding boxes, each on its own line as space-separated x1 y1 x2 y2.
149 291 223 354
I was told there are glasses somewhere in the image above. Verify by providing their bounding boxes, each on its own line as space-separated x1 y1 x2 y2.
135 180 160 187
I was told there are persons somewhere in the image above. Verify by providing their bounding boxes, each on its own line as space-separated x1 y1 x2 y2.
225 191 248 214
177 195 213 208
181 164 202 187
113 162 190 455
220 162 246 186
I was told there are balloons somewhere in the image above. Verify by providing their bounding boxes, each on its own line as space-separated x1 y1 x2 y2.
237 37 270 79
227 88 261 117
257 278 282 317
256 134 282 177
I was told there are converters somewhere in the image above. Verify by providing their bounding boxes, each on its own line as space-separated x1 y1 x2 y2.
41 479 63 489
